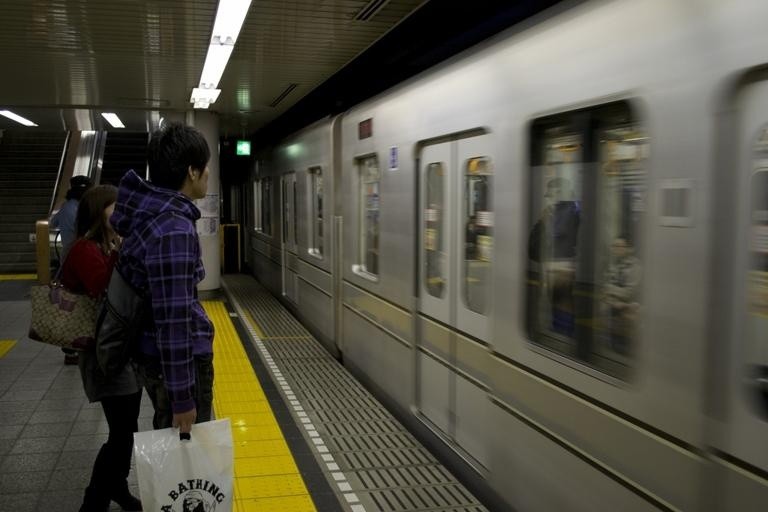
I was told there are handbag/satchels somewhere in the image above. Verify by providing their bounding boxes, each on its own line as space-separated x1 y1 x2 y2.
96 265 147 378
28 242 109 353
132 419 233 512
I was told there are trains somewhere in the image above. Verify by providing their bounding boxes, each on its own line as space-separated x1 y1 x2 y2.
218 0 768 512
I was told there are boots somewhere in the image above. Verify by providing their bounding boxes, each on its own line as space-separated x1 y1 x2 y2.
79 447 118 512
111 449 143 512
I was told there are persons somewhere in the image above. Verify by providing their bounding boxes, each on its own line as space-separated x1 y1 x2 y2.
65 183 142 512
527 181 589 349
608 233 639 346
53 175 93 365
109 121 215 512
